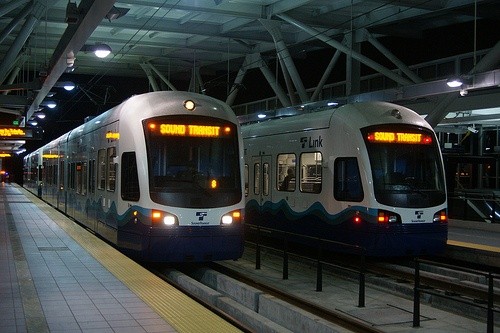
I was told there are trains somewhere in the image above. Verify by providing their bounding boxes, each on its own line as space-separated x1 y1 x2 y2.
234 99 450 267
21 91 246 267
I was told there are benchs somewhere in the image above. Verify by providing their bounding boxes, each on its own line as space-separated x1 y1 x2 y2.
278 176 322 193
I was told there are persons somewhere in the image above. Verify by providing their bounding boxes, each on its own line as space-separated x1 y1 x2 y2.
280 169 294 190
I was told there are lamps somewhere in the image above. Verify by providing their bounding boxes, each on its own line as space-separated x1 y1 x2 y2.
445 77 474 88
78 44 112 59
258 111 275 119
27 121 38 126
33 112 46 119
106 6 120 22
51 82 76 91
38 101 57 109
328 99 348 108
